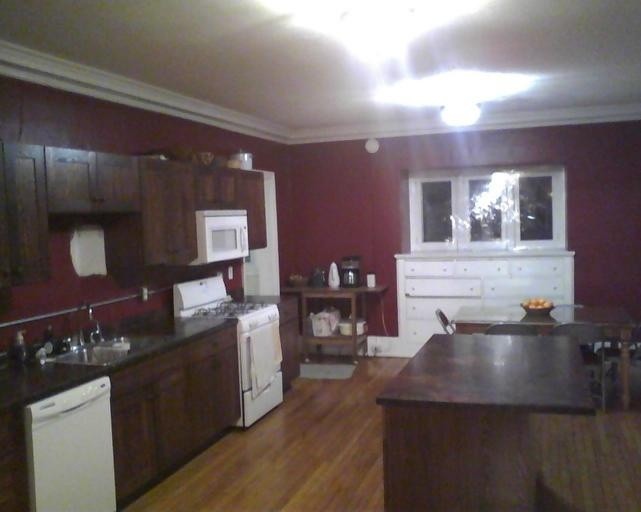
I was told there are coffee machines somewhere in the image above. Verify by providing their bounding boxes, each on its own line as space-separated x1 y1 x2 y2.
341 255 363 287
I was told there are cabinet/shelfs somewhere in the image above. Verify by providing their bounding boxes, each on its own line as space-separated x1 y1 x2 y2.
110 324 241 506
281 283 390 366
396 258 575 358
1 142 268 285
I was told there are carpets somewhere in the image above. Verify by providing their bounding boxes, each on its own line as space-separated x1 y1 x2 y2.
299 363 357 379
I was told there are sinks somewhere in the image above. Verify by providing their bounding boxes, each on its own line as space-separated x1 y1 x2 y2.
44 346 140 366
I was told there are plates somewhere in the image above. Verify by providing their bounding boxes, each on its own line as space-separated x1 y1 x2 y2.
520 303 556 317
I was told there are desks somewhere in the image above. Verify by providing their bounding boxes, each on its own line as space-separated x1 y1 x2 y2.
377 333 593 512
451 305 638 410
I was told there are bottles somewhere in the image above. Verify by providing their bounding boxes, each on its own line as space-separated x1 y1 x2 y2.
327 262 341 288
320 270 326 282
13 324 71 365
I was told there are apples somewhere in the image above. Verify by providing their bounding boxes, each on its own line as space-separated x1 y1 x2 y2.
522 297 552 310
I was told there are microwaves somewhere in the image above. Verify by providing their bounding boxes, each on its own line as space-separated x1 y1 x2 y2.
194 209 250 267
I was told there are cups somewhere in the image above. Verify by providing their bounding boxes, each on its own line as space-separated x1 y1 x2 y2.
140 286 150 302
366 272 376 287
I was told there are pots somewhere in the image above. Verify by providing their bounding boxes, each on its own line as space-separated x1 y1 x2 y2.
338 314 367 336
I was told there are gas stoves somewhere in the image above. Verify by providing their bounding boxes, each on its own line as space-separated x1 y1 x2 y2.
188 299 269 319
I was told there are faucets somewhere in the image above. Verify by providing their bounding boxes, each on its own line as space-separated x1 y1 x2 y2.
62 301 93 350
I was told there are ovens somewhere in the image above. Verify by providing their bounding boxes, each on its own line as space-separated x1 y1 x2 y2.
239 319 285 427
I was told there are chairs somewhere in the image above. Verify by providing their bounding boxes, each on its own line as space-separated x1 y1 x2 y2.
436 308 457 335
484 321 640 414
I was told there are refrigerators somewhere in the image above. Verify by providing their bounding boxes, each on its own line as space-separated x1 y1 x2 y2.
23 376 119 512
